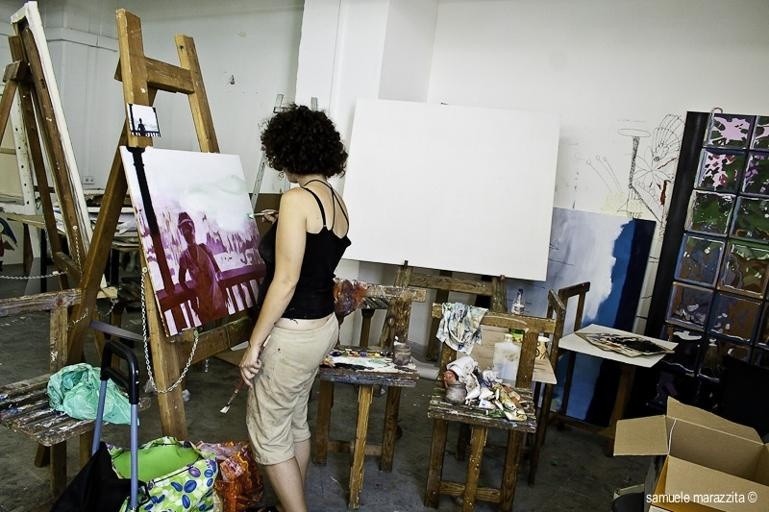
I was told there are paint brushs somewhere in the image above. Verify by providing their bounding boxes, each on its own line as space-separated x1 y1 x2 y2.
219 334 272 414
249 211 279 216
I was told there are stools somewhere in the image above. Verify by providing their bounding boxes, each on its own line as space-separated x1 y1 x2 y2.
1 372 151 498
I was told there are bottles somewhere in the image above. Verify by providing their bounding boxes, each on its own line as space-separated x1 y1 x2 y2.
510 288 526 316
493 334 521 383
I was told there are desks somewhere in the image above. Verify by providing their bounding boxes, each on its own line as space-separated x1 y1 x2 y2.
1 210 140 326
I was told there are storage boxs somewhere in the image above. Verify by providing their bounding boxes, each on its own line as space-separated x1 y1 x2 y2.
644 498 674 512
612 396 769 512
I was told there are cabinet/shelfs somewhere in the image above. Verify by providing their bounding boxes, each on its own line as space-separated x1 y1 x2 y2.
424 302 557 509
313 279 427 510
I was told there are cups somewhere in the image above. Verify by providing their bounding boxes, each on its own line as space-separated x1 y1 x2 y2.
445 382 467 405
393 343 412 366
534 335 551 361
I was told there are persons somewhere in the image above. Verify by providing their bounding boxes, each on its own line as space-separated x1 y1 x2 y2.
138 117 146 136
239 102 351 512
176 212 232 330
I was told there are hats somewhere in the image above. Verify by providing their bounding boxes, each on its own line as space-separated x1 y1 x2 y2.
177 212 193 226
